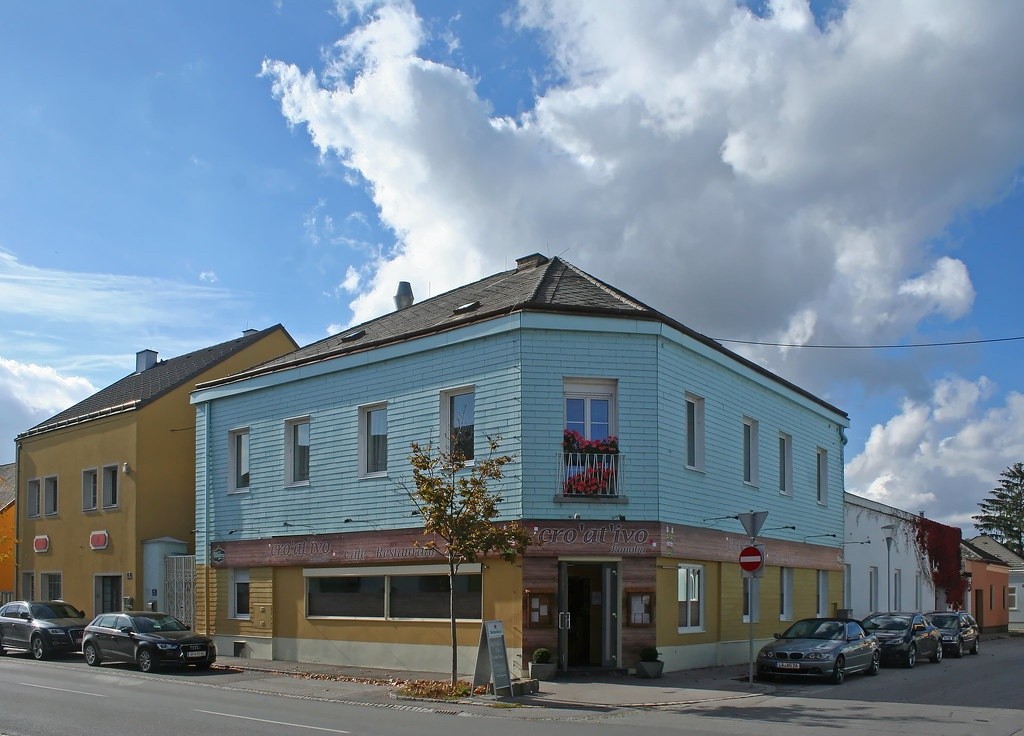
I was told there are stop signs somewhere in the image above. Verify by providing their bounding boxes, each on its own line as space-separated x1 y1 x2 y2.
739 546 763 573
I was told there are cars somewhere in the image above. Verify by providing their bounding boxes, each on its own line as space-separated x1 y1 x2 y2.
857 612 943 668
80 610 216 674
923 609 981 658
759 617 882 687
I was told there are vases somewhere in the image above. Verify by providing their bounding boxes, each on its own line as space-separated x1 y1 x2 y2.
569 454 610 463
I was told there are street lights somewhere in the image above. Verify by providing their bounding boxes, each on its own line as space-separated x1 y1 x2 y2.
881 523 900 614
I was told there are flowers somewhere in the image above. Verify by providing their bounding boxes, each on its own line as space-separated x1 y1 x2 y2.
563 428 619 493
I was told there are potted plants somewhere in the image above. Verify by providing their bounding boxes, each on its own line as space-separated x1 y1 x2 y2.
639 647 665 677
528 648 556 680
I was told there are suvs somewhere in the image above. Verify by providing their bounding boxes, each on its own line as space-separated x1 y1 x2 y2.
0 600 95 661
